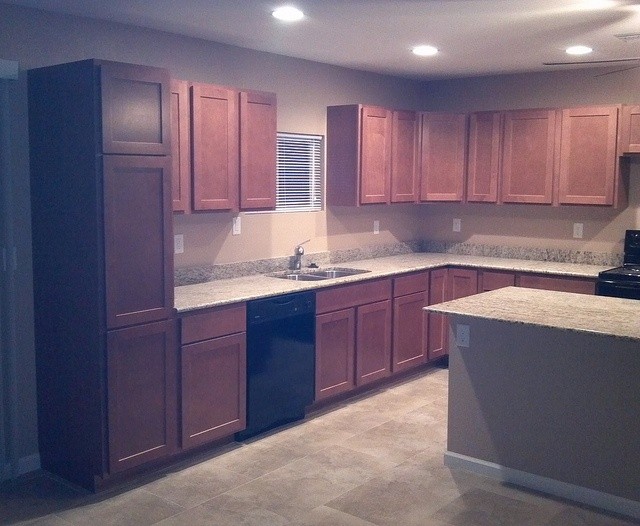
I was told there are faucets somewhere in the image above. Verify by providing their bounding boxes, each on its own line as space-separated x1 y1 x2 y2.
295 238 310 269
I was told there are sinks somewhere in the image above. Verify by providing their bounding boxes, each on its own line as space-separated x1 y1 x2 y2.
264 267 372 281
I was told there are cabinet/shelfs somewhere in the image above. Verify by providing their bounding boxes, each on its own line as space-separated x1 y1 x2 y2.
502 104 620 207
520 274 596 296
327 103 416 204
419 111 499 203
448 268 478 300
170 78 277 213
429 267 448 360
315 280 391 405
617 105 640 153
178 303 247 454
482 272 515 290
26 60 179 494
392 271 429 372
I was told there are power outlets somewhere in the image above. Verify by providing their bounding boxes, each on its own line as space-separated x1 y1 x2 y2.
374 220 380 234
174 234 185 253
573 222 584 238
452 218 461 233
